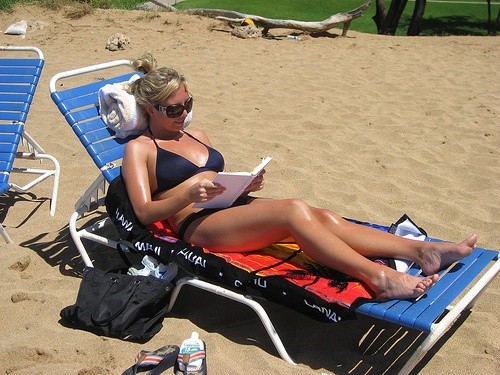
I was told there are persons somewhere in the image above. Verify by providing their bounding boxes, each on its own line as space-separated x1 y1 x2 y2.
122 50 477 301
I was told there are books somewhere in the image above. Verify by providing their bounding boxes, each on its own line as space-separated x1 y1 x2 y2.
192 157 273 208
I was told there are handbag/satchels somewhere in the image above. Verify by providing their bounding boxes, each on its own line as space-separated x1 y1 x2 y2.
59 240 176 344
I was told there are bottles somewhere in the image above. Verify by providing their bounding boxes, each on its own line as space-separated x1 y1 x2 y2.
288 35 300 40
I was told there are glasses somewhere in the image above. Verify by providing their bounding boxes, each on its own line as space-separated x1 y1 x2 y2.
153 97 194 119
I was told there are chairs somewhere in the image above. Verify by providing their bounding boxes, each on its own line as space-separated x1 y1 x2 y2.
0 45 61 245
48 60 500 375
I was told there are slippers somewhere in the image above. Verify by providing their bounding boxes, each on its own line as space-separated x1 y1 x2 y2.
121 344 180 375
174 339 207 375
